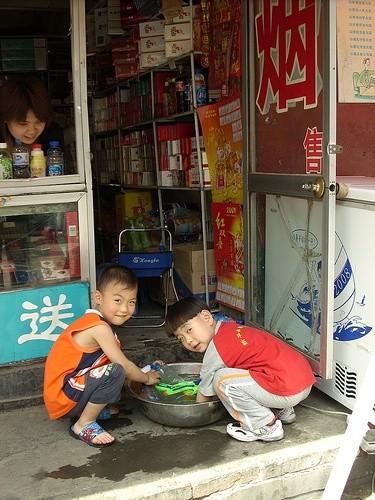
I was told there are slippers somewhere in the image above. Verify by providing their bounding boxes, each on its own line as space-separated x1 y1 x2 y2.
71 405 111 420
70 423 114 447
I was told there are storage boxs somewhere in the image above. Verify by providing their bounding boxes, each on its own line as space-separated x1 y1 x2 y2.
85 0 219 303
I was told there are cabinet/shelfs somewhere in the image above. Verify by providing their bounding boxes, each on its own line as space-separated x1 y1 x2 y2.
90 50 221 313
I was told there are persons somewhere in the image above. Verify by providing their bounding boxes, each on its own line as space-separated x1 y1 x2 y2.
0 73 66 157
165 295 316 442
43 265 163 448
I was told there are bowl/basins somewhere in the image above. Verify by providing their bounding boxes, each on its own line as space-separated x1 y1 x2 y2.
128 362 228 428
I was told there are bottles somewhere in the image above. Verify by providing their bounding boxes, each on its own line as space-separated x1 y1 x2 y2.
163 62 216 117
12 139 30 178
152 363 185 385
214 312 244 325
47 141 65 176
124 202 201 252
30 144 46 177
0 143 13 179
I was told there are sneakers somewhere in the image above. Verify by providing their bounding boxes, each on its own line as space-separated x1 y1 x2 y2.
271 406 296 425
227 419 284 442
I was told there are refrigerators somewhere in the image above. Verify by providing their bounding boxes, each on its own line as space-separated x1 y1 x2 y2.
266 176 375 414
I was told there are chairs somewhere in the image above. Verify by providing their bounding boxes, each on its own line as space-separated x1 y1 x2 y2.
112 228 180 328
